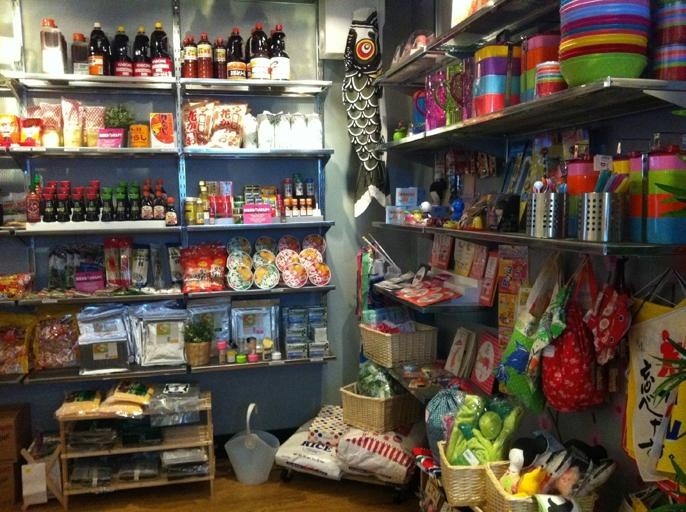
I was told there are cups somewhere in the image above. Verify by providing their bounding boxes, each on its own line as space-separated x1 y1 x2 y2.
413 57 474 130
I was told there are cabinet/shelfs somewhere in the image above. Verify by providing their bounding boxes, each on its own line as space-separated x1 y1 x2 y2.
0 1 336 386
21 390 215 511
371 0 686 490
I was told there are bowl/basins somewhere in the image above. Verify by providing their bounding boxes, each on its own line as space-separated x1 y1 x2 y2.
558 1 651 88
217 342 226 365
536 61 568 96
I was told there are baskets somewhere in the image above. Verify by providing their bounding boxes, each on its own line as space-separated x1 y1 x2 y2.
438 440 486 507
359 321 439 369
485 462 598 512
341 381 426 433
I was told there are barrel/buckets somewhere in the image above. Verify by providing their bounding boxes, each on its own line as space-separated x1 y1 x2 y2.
226 27 247 79
40 16 68 74
267 29 275 43
112 27 133 76
249 22 273 79
150 22 173 77
87 23 113 75
133 26 153 77
245 27 255 57
269 24 291 80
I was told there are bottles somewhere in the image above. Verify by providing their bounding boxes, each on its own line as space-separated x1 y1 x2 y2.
184 181 210 225
283 173 315 217
26 178 179 226
181 31 226 78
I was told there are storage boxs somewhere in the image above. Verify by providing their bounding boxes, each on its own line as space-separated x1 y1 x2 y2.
0 461 20 512
0 405 33 462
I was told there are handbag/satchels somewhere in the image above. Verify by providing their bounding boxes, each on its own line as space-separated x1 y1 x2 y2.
543 288 611 414
496 305 567 415
